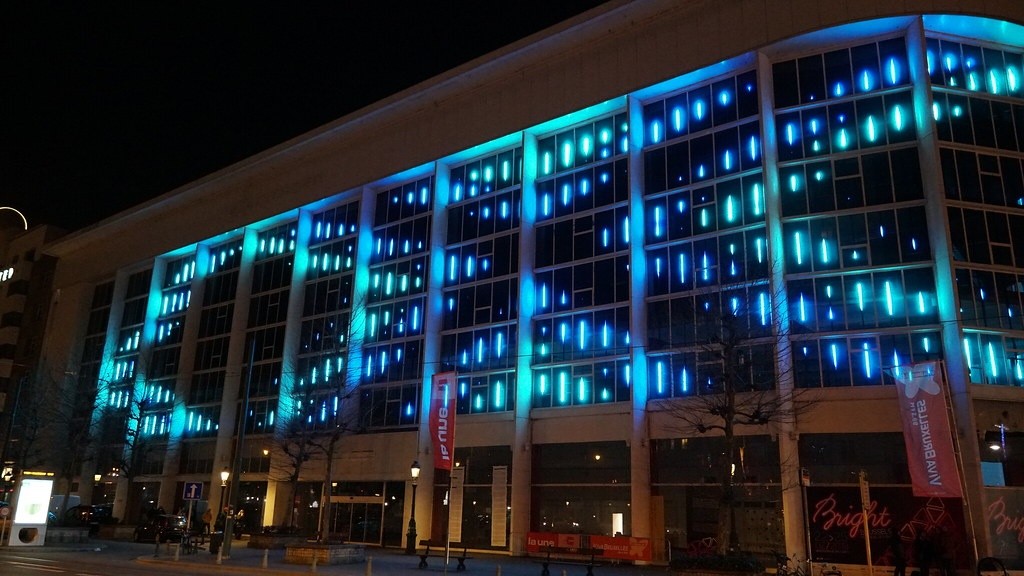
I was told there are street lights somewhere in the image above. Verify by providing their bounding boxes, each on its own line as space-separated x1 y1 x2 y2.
405 461 421 554
208 466 231 556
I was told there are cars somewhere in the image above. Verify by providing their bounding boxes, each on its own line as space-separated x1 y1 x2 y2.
57 506 107 534
133 514 188 542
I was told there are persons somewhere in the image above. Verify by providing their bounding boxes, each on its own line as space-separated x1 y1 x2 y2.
214 506 228 531
199 508 213 537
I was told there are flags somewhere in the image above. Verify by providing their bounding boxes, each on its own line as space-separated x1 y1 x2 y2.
890 359 963 498
428 370 456 471
